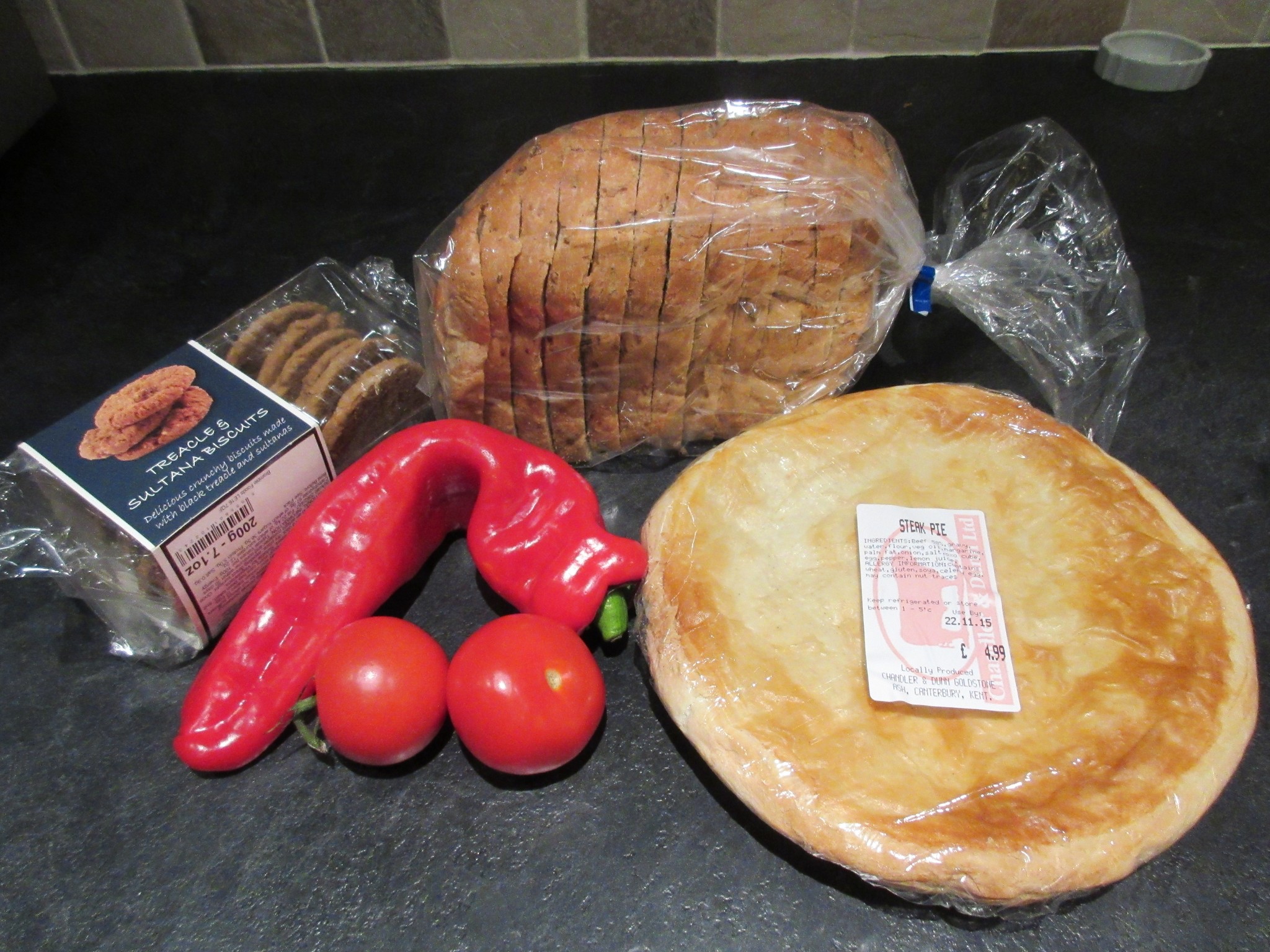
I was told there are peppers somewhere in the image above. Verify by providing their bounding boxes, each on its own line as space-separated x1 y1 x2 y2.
172 418 647 773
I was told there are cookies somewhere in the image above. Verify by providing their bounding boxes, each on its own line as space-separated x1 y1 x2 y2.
224 301 427 468
78 365 213 461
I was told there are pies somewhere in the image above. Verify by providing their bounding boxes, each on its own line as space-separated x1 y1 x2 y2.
626 378 1260 912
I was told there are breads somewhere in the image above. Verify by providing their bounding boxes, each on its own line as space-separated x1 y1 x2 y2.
433 105 893 462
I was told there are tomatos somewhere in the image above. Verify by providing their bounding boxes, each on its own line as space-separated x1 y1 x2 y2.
312 617 449 768
445 613 606 776
